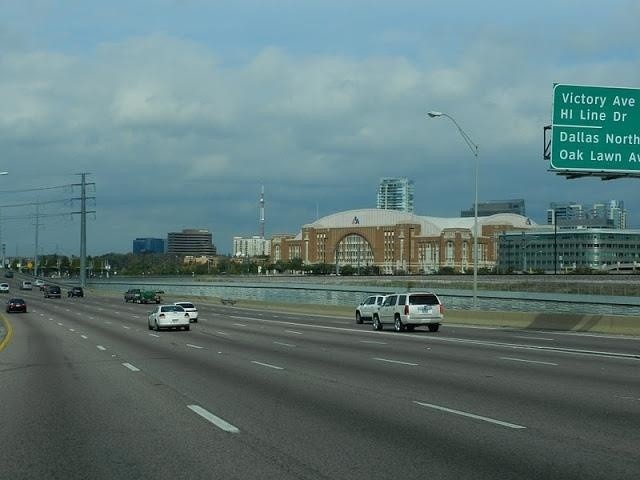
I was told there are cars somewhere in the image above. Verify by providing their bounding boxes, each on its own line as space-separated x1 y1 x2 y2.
355 291 445 332
124 287 199 331
0 270 83 313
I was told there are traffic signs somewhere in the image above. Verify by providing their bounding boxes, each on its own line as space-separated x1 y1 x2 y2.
550 84 640 173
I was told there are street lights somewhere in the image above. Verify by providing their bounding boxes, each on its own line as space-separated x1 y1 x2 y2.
0 171 8 175
427 110 477 311
